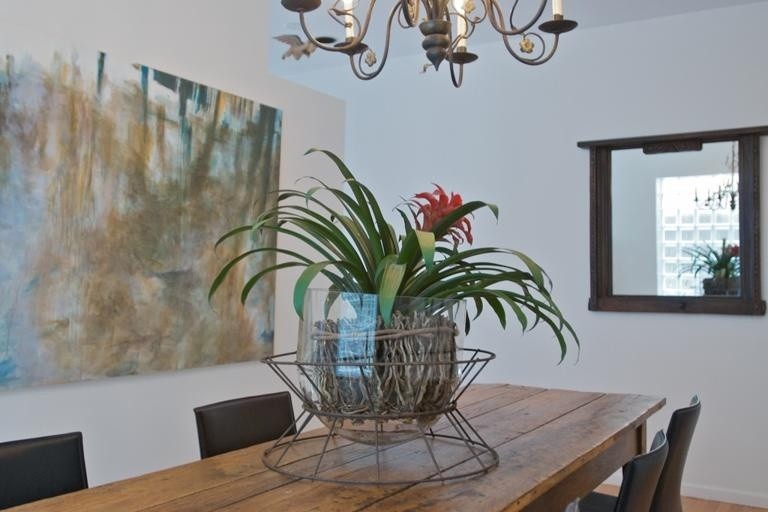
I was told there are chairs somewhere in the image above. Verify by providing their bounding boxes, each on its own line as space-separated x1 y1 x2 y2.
576 379 701 511
1 430 93 511
190 390 297 457
621 429 670 510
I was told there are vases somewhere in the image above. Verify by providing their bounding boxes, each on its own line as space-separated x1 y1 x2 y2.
704 278 745 298
296 289 460 446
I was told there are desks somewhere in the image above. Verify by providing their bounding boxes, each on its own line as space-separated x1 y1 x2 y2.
8 380 666 512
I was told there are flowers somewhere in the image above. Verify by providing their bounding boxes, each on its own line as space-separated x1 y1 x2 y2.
676 238 742 279
209 147 583 406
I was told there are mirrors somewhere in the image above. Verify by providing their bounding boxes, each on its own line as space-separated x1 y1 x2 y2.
576 125 764 318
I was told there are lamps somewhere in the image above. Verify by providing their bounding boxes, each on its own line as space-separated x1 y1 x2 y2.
274 1 578 89
